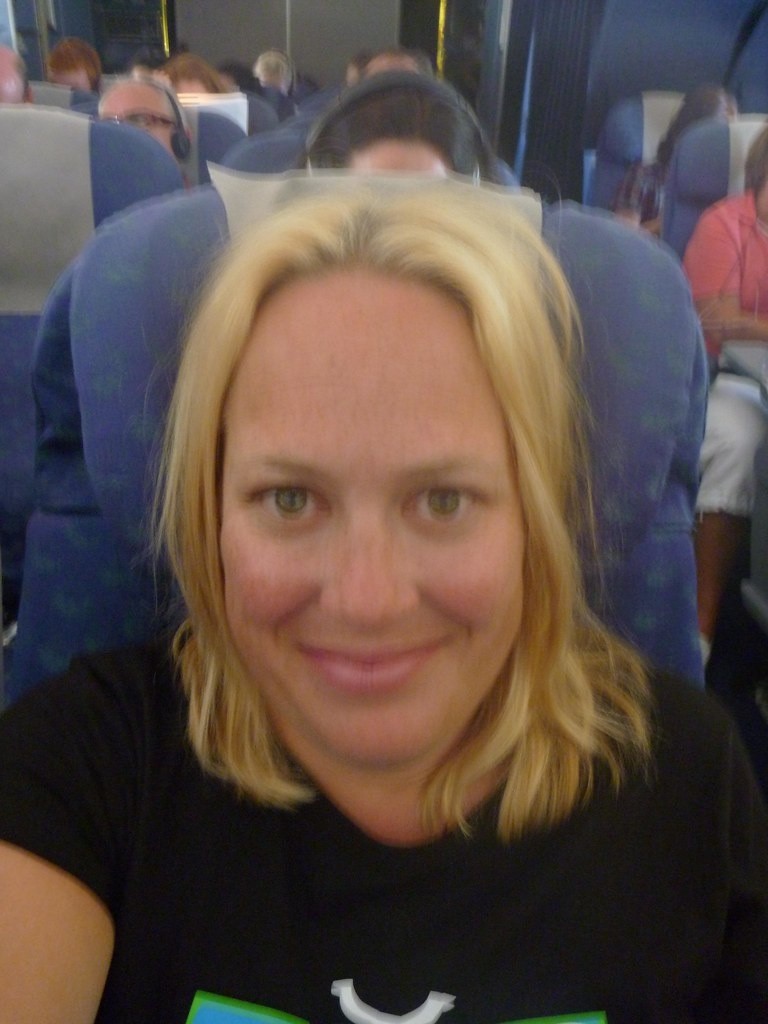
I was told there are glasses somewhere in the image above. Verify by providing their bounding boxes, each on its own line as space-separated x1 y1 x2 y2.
102 111 175 132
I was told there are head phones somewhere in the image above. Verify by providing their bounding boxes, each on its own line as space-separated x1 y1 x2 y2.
164 91 191 160
303 73 496 205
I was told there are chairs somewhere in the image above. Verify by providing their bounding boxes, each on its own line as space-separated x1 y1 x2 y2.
1 37 768 708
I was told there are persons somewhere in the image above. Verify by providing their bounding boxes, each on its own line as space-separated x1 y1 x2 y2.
1 183 768 1024
0 36 767 664
